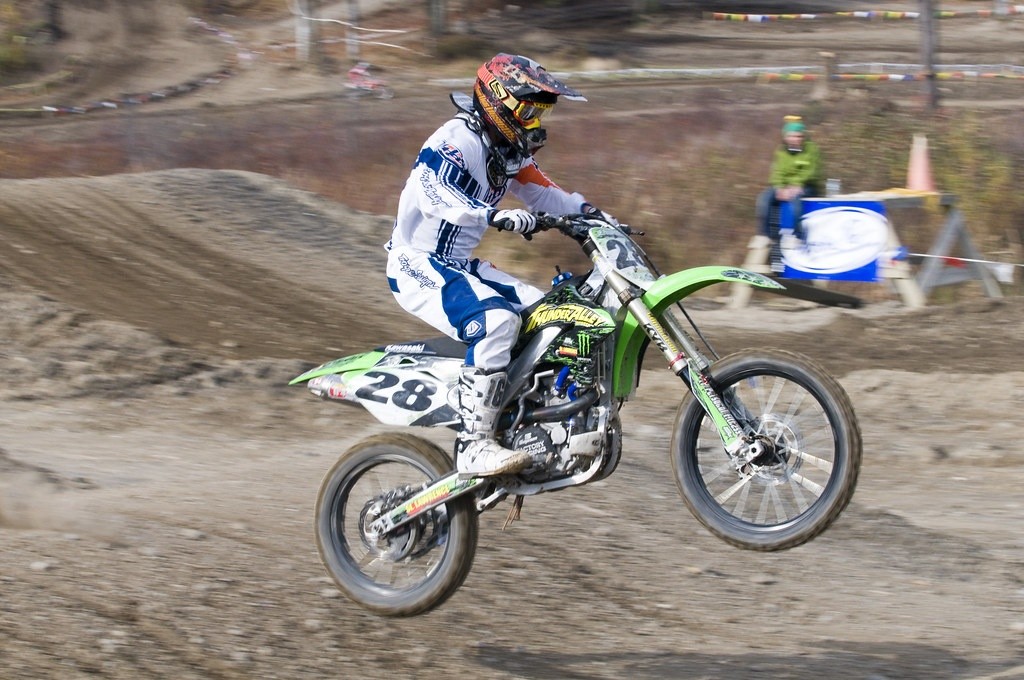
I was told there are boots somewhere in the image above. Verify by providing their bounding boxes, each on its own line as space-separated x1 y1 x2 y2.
452 362 533 481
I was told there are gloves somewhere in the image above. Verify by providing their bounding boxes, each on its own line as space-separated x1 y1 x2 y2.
591 206 620 232
491 208 536 235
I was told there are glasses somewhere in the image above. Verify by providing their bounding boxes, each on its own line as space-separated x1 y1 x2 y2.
513 100 556 127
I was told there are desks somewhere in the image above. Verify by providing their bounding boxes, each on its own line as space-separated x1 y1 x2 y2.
720 188 1024 314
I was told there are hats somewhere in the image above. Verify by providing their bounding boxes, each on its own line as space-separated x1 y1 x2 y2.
780 121 808 136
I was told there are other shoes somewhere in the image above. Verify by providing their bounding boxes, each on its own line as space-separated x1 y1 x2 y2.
746 234 774 250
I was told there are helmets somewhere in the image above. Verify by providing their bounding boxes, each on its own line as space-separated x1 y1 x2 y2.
473 51 582 159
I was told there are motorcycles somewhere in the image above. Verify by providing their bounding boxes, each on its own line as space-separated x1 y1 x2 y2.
286 206 866 619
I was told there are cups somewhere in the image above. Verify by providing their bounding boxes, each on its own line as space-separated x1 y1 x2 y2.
825 178 842 198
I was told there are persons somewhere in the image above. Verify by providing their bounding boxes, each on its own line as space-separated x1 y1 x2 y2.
386 52 620 478
754 118 820 273
